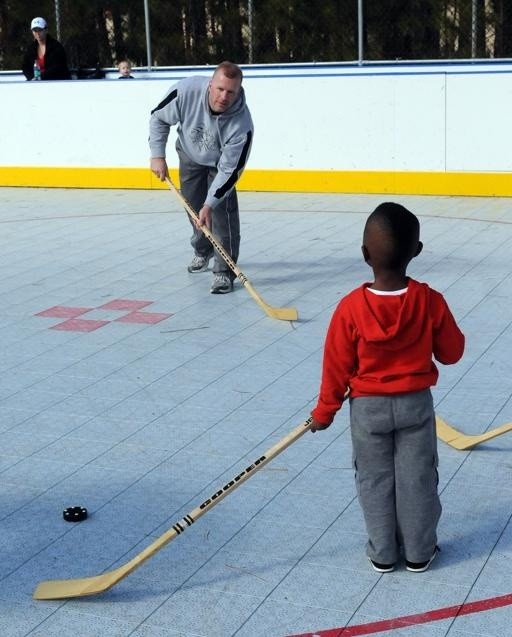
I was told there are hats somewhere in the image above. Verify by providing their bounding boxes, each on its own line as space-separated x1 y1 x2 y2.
31 17 47 30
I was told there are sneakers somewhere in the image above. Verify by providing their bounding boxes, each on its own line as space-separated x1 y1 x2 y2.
188 252 214 272
210 275 232 293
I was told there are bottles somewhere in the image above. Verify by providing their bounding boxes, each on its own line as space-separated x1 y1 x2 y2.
32 58 41 80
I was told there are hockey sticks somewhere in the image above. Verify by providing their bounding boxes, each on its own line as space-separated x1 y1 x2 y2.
34 418 317 599
434 414 512 451
166 176 299 320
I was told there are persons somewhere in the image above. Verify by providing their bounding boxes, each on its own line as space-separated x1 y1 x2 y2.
21 17 72 81
118 60 134 79
310 203 465 572
148 61 254 293
